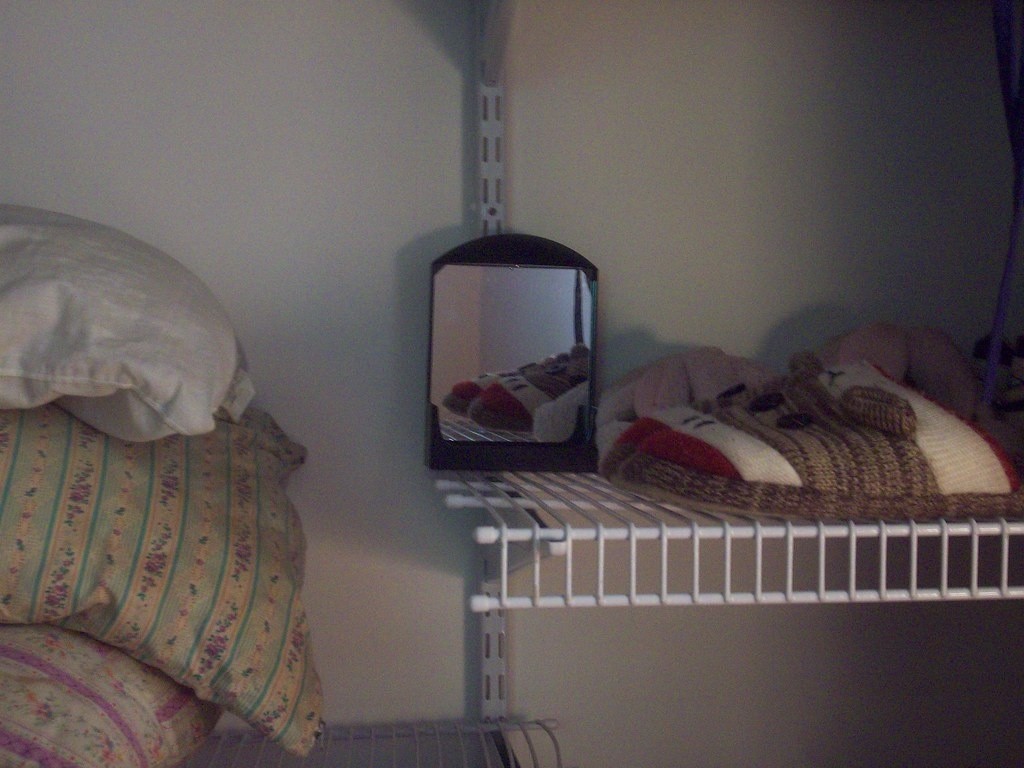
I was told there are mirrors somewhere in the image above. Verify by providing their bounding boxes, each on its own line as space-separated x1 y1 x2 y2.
425 228 599 474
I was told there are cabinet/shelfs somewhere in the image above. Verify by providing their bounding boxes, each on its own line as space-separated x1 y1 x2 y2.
176 472 1024 768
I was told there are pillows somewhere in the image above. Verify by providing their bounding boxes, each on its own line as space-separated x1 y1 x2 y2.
1 201 252 443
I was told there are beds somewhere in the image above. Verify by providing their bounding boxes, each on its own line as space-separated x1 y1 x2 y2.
0 415 307 768
